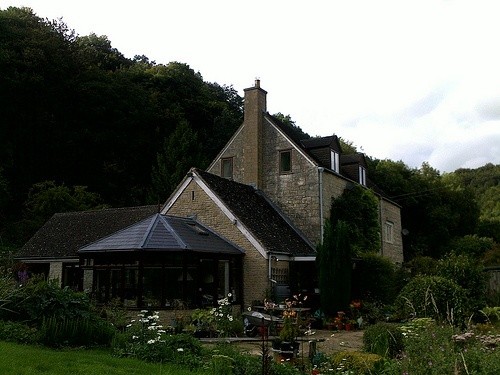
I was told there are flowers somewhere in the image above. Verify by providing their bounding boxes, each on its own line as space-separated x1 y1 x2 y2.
264 294 308 343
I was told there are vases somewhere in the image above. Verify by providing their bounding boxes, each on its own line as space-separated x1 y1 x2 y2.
273 341 300 364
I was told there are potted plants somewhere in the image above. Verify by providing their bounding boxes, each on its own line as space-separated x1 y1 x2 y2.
326 311 356 331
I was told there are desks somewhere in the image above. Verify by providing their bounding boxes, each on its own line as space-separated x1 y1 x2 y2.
254 305 310 326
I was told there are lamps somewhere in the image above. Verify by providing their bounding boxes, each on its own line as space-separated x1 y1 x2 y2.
271 255 279 263
